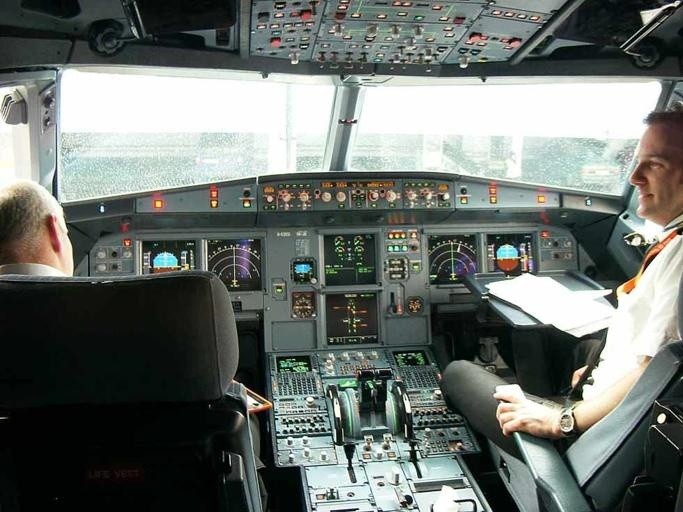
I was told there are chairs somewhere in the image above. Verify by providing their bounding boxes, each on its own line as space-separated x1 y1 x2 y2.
0 270 264 512
484 341 681 512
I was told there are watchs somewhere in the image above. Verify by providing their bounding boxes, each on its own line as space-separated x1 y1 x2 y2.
559 406 577 439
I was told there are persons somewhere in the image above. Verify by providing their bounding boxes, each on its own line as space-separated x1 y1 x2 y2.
440 106 682 461
0 180 75 277
